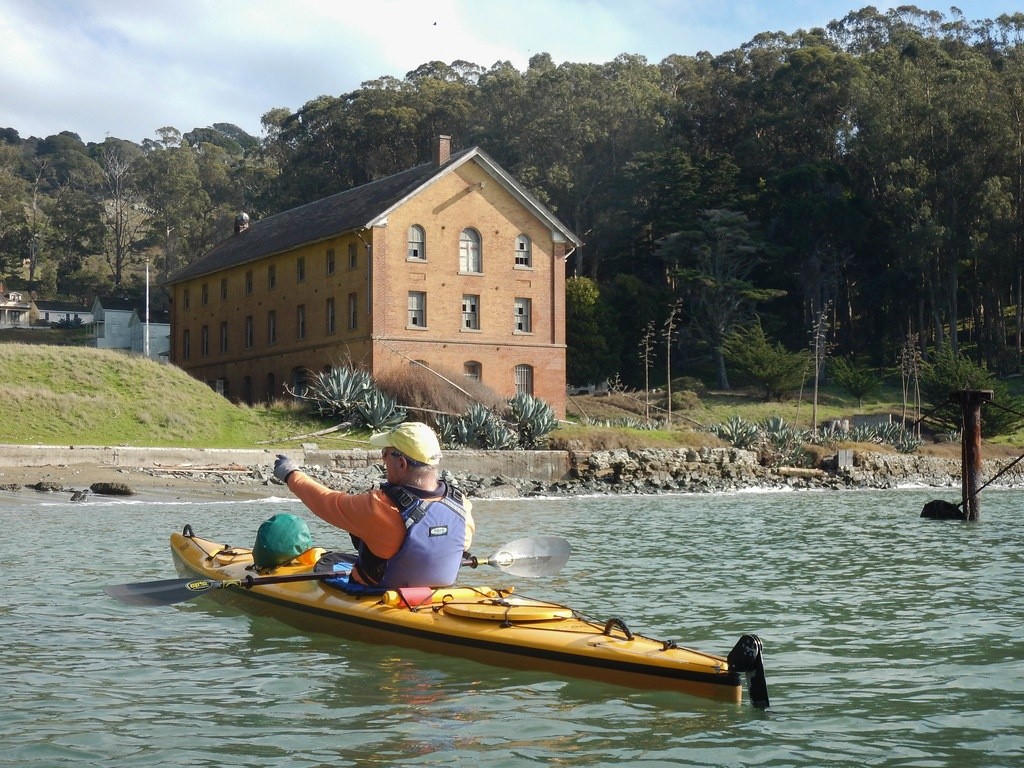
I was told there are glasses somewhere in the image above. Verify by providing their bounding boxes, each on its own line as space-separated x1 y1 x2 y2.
381 448 416 464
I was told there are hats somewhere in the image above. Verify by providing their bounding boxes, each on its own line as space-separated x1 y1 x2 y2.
369 422 441 465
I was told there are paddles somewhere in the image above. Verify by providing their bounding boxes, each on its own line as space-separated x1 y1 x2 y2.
102 536 572 623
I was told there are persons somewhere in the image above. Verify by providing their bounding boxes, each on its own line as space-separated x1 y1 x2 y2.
272 422 477 589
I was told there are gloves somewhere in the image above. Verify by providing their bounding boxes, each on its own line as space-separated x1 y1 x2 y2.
272 454 302 483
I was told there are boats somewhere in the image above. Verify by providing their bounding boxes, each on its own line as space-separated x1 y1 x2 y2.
168 523 773 714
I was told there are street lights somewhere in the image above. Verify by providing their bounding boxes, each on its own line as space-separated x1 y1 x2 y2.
144 258 150 359
